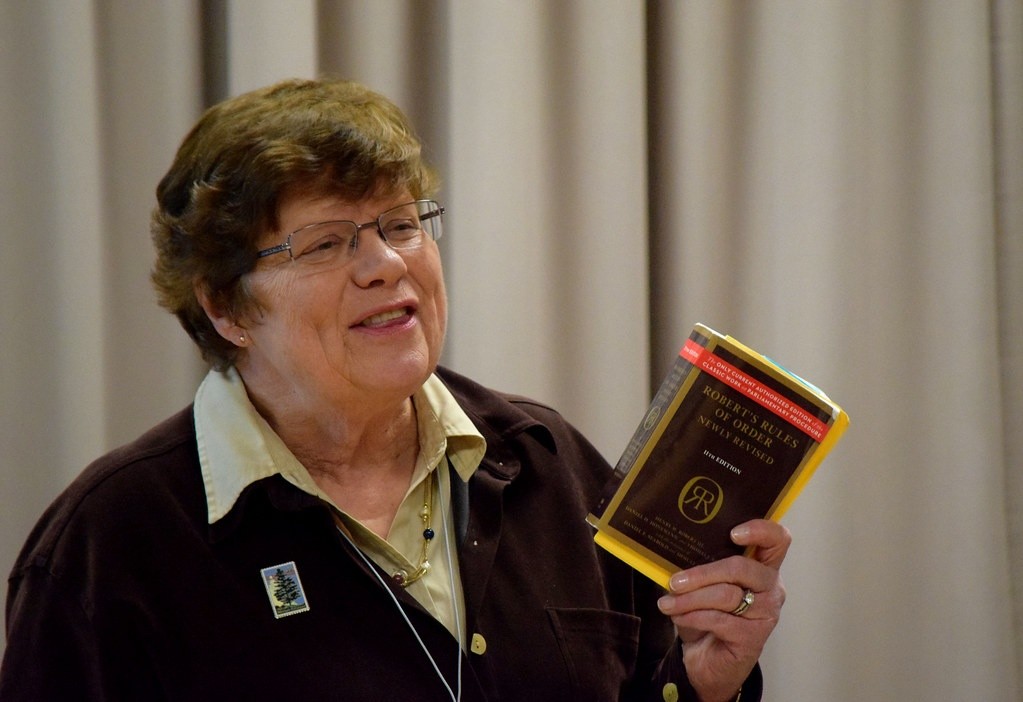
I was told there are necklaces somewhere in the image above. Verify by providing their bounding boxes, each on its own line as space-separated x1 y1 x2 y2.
347 413 449 595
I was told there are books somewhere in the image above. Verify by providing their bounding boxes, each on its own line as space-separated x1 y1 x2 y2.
583 318 854 603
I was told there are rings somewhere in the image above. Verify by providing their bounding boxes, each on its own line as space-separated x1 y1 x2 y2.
730 588 757 617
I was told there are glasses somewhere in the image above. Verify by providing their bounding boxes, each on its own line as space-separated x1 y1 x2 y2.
224 200 446 280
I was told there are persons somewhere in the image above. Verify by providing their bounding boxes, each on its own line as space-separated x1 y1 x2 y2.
1 73 791 702
